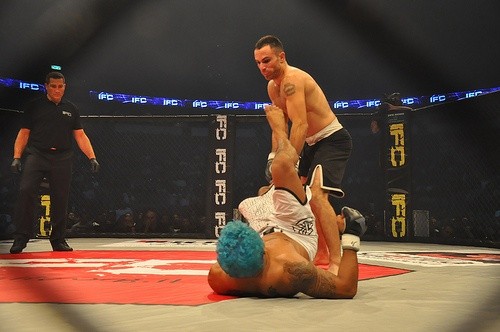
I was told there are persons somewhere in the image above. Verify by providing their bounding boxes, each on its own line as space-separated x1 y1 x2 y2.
369 90 414 167
0 208 205 235
363 199 500 242
9 72 100 254
207 103 368 300
253 34 352 275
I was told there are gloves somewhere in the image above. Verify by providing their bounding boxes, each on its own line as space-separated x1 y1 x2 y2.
90 159 98 176
340 207 365 250
10 159 22 172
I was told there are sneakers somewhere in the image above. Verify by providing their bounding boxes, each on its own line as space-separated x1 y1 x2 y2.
10 240 27 253
50 237 73 251
